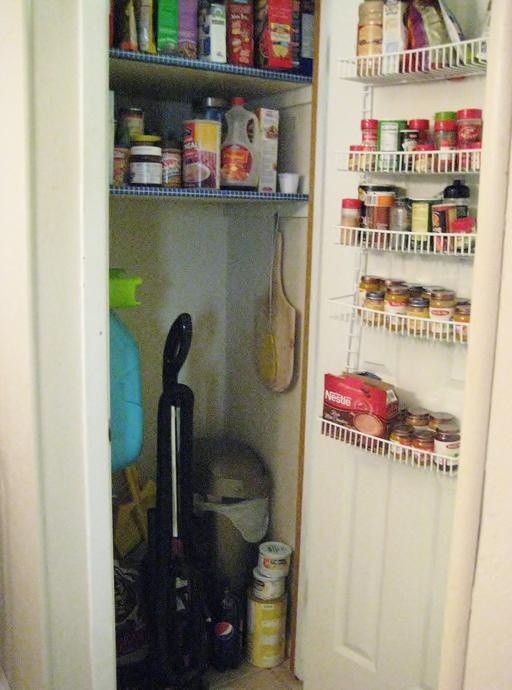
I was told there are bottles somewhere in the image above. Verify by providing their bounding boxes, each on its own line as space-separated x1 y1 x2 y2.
246 585 288 669
339 183 408 250
226 1 254 67
357 274 470 341
252 566 286 601
254 105 279 194
255 0 292 69
430 179 476 250
349 108 482 170
114 96 260 189
357 0 384 27
357 24 383 76
199 4 226 64
257 540 292 578
388 406 460 470
410 198 443 250
212 586 244 674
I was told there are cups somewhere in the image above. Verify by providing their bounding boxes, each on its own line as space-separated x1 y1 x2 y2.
277 172 300 195
